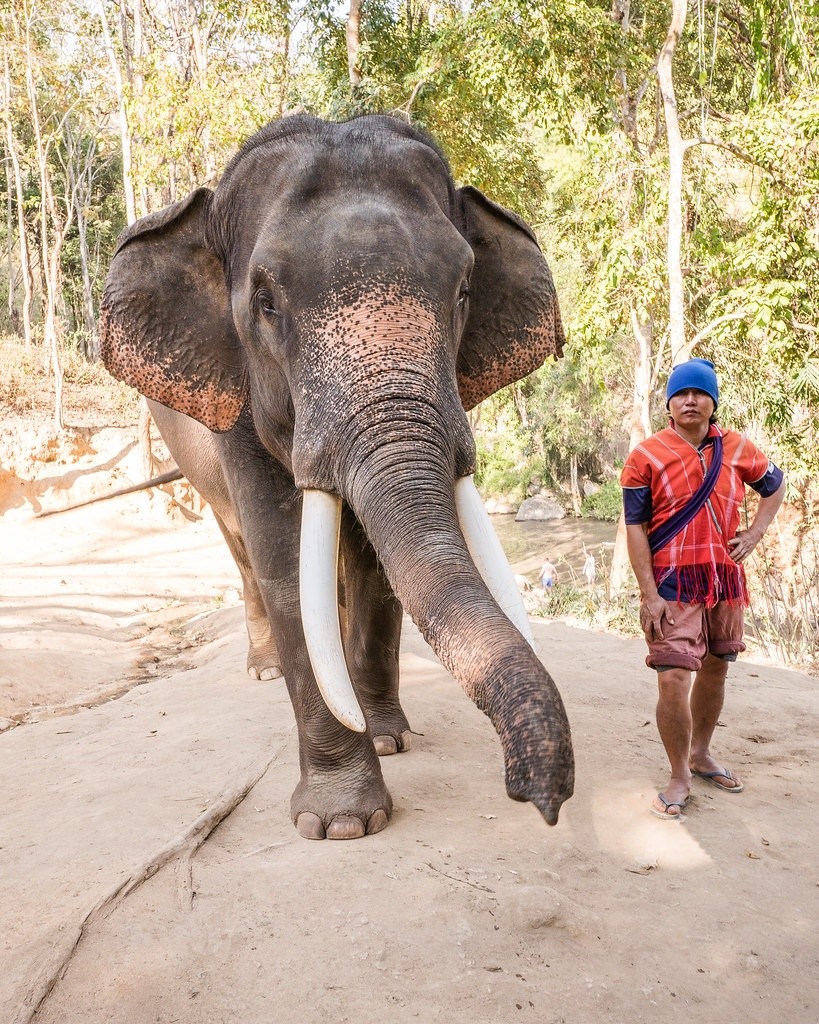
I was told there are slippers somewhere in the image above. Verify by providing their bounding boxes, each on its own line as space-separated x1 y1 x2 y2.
690 767 743 793
649 792 690 820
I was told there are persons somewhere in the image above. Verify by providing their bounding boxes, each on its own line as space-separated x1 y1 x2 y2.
537 558 558 596
620 357 784 820
583 551 596 586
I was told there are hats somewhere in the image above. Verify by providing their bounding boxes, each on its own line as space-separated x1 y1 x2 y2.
665 357 718 413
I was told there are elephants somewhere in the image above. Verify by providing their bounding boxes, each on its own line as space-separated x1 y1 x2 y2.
96 112 577 841
